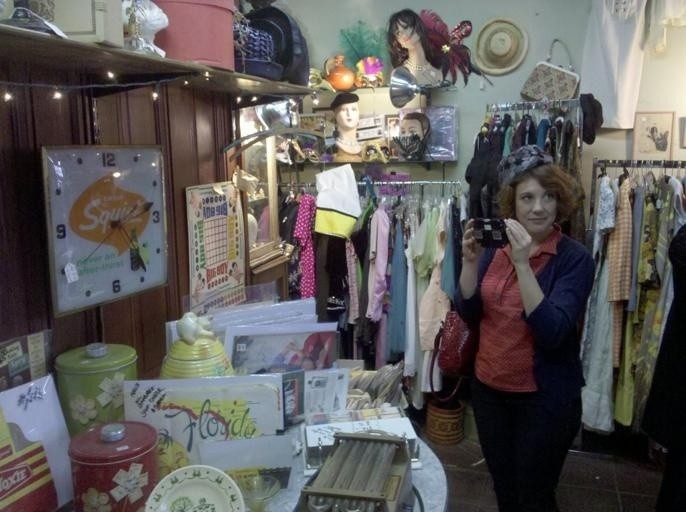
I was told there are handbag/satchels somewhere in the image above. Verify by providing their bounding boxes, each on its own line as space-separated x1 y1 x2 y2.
520 38 580 114
429 310 477 402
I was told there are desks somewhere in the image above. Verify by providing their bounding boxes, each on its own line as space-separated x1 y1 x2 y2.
248 421 449 512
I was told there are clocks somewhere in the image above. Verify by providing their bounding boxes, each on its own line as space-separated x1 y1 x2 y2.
41 144 169 318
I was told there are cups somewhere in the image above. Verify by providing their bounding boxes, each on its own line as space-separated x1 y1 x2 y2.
238 475 281 512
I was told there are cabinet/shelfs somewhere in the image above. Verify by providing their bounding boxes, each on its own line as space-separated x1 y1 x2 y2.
277 86 457 172
232 106 290 304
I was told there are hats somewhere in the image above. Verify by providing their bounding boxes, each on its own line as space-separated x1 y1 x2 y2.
497 144 554 189
329 93 360 107
473 18 529 75
233 7 310 85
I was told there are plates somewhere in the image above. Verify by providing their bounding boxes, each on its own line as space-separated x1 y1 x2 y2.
144 464 245 512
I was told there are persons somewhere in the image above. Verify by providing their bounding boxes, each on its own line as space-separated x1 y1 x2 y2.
330 93 364 158
387 9 446 88
401 112 430 143
453 145 596 512
640 224 686 510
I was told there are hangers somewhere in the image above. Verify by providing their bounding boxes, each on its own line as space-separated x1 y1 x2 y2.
490 100 581 148
222 121 324 162
281 180 462 219
597 159 685 192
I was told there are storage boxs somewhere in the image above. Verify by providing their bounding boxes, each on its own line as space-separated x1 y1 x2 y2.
151 0 235 72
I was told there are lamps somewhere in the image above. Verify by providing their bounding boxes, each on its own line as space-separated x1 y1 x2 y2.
389 66 431 108
255 99 301 141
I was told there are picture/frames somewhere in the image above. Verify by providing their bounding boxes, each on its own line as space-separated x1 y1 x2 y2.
632 111 674 161
